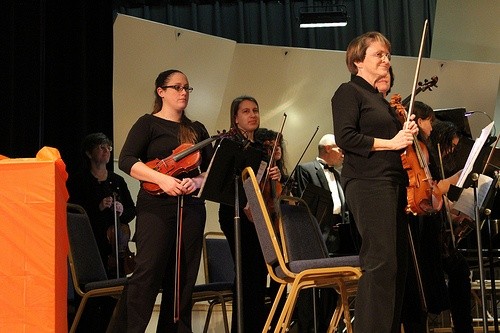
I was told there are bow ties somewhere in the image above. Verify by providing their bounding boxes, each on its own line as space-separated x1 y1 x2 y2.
320 162 335 173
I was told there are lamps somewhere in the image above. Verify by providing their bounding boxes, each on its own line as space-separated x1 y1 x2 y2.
292 0 350 29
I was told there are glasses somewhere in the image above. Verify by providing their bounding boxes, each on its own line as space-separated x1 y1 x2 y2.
161 85 194 93
97 145 112 151
364 52 391 61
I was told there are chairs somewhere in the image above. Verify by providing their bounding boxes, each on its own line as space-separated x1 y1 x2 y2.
66 203 165 333
201 232 235 333
241 166 364 333
190 282 235 333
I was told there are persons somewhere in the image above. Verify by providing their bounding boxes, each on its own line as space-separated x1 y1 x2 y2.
213 96 290 333
293 134 354 332
69 133 137 333
118 70 215 333
404 102 472 333
332 32 419 333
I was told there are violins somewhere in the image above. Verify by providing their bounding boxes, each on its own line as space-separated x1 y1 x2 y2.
391 92 443 217
139 127 238 196
242 140 290 233
106 187 135 274
401 75 439 105
